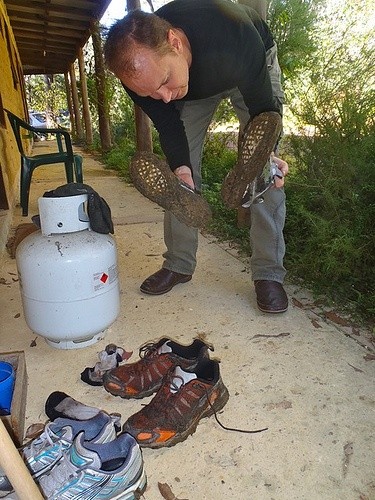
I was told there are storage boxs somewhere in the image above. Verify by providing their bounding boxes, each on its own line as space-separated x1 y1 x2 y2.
0 351 28 445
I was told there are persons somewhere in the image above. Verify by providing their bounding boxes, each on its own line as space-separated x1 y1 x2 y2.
104 0 289 312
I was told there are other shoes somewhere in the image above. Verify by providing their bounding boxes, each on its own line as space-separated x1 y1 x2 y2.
130 151 213 229
221 111 283 212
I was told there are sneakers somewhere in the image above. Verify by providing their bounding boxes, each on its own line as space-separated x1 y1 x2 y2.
0 410 117 492
0 432 147 500
122 361 268 450
104 338 214 401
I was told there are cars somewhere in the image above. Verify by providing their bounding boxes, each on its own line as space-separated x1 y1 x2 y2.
28 112 53 141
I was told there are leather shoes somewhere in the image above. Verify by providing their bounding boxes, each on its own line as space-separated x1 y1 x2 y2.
140 268 192 295
254 279 288 314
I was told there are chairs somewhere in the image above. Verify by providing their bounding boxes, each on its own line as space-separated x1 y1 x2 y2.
3 108 83 217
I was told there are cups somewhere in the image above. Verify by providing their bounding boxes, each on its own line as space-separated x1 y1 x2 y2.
0 360 16 414
